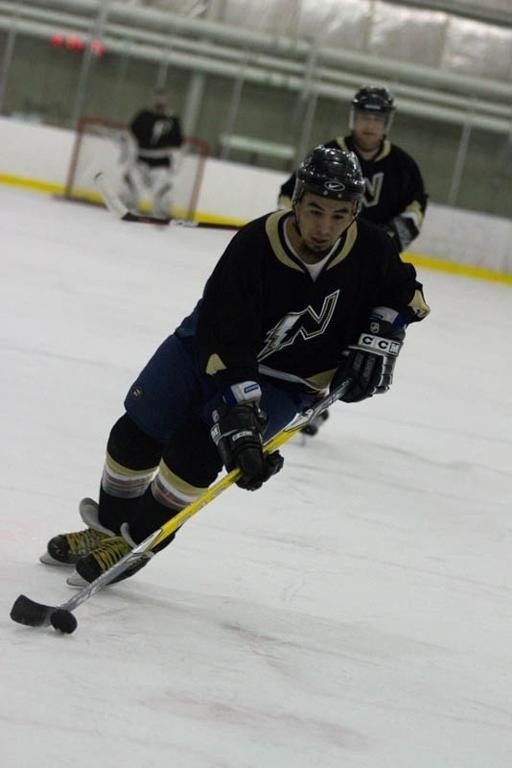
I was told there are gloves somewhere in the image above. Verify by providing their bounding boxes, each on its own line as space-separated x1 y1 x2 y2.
329 320 404 402
211 403 283 490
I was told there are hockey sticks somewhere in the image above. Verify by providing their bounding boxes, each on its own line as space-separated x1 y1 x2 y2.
11 376 352 630
95 172 244 232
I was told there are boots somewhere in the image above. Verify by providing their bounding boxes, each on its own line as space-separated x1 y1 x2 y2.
47 498 154 585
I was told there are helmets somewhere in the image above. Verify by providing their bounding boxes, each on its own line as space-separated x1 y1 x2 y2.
291 147 365 217
350 85 394 135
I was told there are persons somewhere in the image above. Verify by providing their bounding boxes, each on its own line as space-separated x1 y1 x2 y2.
47 145 431 586
115 85 183 221
278 84 429 254
